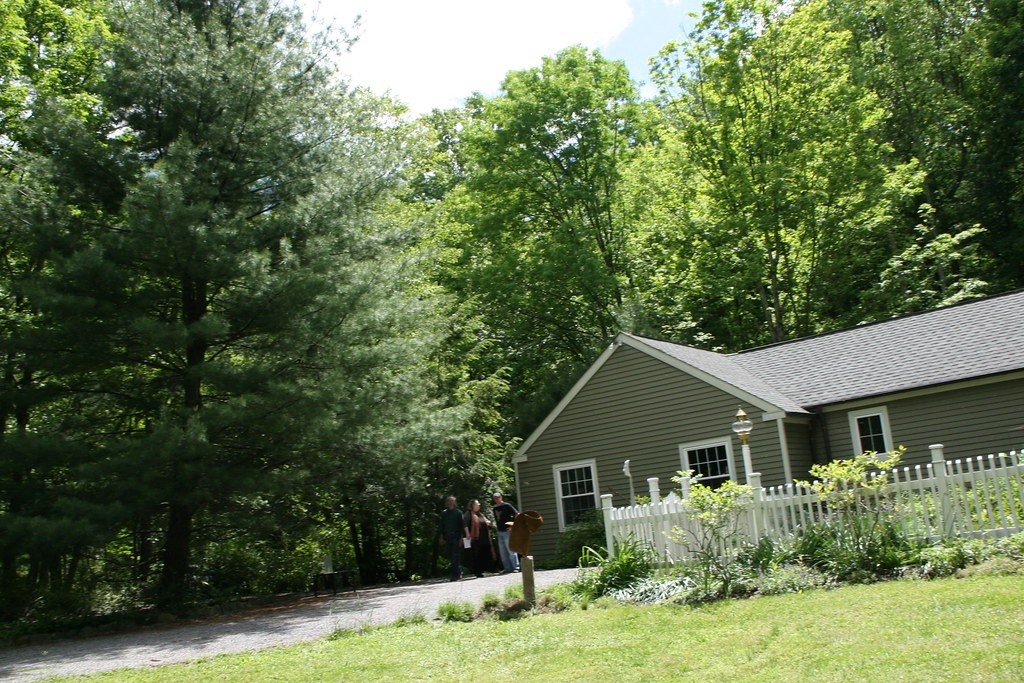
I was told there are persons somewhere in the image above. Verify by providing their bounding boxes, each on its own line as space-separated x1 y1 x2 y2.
439 496 464 582
463 492 521 578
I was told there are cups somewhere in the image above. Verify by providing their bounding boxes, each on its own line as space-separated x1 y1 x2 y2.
463 537 471 548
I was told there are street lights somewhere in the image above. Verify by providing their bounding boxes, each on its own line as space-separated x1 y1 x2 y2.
731 405 755 490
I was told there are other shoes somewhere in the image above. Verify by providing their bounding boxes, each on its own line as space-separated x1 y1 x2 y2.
450 575 460 582
477 573 484 577
513 568 521 573
499 570 510 574
